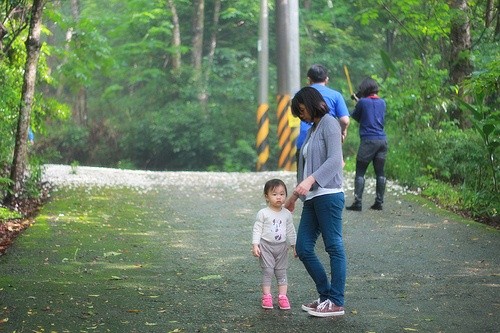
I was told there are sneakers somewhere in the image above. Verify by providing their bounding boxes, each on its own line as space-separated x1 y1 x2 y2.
262 293 274 308
278 294 290 309
308 299 345 317
302 297 321 311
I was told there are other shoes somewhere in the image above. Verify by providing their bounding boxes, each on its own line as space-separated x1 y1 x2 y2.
346 204 362 210
371 203 382 210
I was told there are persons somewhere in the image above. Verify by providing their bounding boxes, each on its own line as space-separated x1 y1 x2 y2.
296 64 350 180
291 86 346 317
346 77 387 212
252 178 298 310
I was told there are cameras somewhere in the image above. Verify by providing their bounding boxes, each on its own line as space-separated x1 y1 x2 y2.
351 93 363 101
